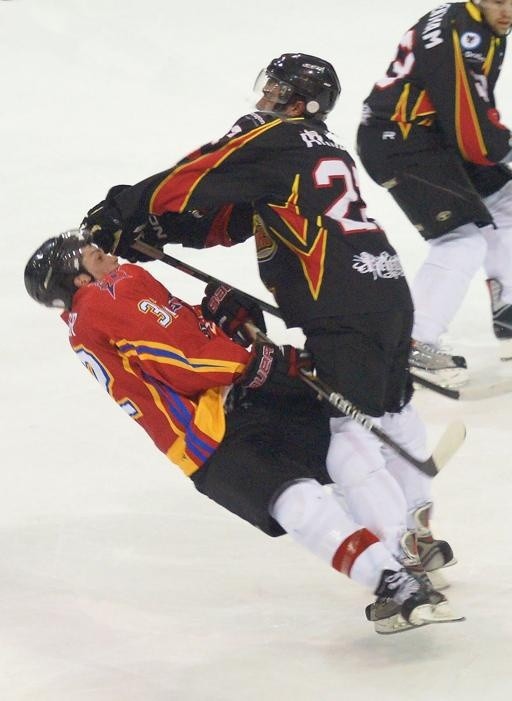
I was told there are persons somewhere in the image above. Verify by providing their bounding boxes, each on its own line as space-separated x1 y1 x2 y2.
356 0 512 391
76 52 458 621
24 231 466 635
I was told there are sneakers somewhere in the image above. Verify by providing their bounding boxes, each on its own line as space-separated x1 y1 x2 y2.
365 502 453 620
408 338 466 371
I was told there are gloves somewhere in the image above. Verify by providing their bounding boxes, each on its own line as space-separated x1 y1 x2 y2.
82 201 163 262
241 342 312 396
202 283 266 346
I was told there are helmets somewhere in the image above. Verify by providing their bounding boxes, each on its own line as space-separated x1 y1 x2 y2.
25 229 93 309
253 53 341 117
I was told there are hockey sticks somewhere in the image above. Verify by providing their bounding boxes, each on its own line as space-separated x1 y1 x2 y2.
243 320 466 480
134 238 511 401
